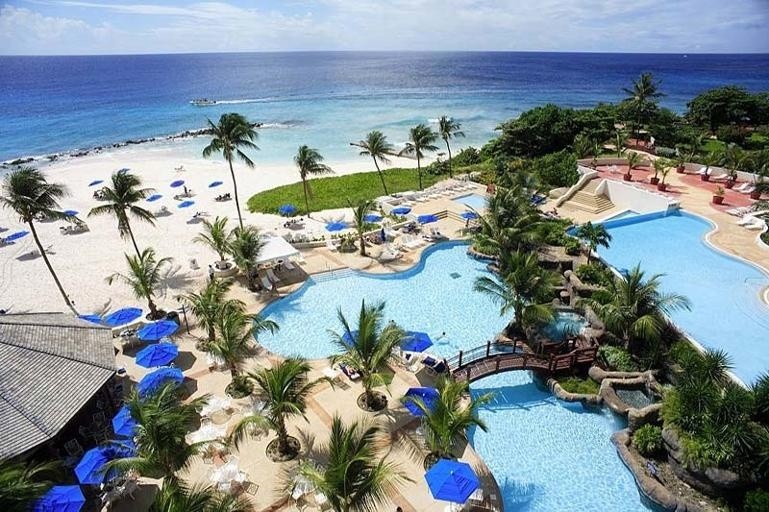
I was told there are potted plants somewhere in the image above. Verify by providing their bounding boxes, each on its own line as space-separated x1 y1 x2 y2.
658 161 676 191
724 150 756 188
676 151 692 173
624 152 643 180
701 150 723 181
751 182 769 200
648 156 666 185
713 186 725 204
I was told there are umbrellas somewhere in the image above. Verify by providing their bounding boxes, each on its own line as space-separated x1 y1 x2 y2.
400 387 442 416
76 315 101 323
278 204 479 232
136 319 179 341
135 341 178 369
4 168 223 241
74 439 136 484
112 406 139 437
33 485 86 512
424 459 481 504
102 307 143 328
399 331 434 353
341 330 360 347
137 367 184 397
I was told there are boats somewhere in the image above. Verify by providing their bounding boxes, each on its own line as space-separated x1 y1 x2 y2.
186 96 216 108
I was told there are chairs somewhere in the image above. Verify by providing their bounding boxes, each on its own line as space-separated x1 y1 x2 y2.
254 181 479 291
63 324 175 512
0 189 231 261
